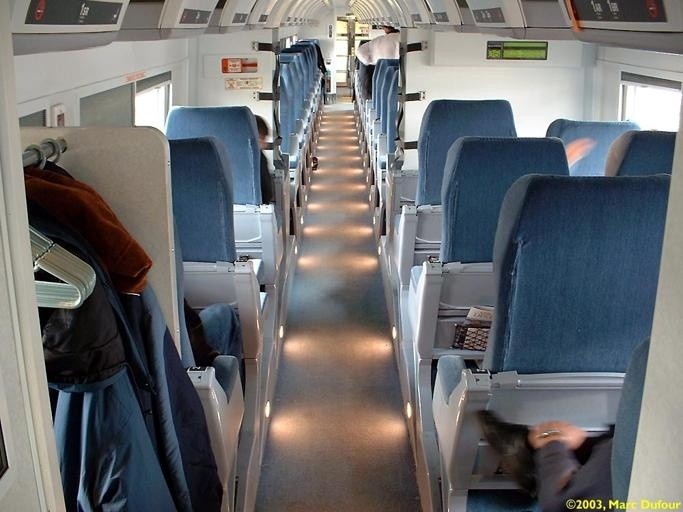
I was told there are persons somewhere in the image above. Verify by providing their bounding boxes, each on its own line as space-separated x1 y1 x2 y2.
474 407 617 512
381 26 400 34
254 114 273 204
183 297 244 376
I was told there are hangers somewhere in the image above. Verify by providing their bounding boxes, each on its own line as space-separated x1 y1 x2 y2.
28 228 95 309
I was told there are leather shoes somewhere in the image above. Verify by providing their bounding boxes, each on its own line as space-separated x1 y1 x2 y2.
477 409 532 486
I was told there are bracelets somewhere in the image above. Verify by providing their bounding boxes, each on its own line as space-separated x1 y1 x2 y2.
532 426 563 440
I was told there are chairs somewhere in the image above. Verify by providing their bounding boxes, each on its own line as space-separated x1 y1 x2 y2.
164 38 674 512
